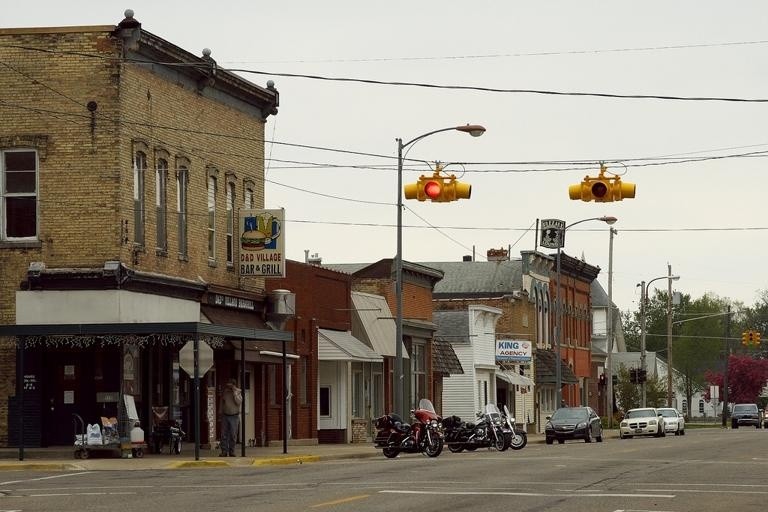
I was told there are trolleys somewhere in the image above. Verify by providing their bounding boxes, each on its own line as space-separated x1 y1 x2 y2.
69 411 150 459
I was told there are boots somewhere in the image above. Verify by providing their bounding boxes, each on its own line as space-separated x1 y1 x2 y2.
219 449 236 457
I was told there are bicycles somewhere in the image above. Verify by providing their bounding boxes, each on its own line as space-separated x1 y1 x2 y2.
167 424 184 454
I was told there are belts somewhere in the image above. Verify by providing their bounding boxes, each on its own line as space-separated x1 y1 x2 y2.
225 414 239 416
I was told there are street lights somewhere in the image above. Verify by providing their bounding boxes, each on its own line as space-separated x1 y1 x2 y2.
393 122 487 416
552 215 620 406
639 274 683 406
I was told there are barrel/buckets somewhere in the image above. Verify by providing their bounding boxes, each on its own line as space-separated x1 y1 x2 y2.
266 288 296 320
130 427 145 444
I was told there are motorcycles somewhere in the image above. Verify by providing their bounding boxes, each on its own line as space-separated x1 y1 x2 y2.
370 396 448 458
436 402 513 452
501 403 533 448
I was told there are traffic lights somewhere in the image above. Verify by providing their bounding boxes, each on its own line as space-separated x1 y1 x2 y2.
748 330 755 346
580 166 623 204
599 374 605 384
613 176 637 200
568 182 588 201
405 179 424 203
754 332 761 345
418 175 455 203
741 332 748 345
446 179 474 202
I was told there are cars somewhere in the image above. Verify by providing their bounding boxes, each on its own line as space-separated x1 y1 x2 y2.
654 406 689 437
619 405 667 438
763 403 767 428
730 403 764 429
544 404 605 444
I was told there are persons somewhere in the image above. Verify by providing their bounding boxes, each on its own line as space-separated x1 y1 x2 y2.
217 377 243 459
